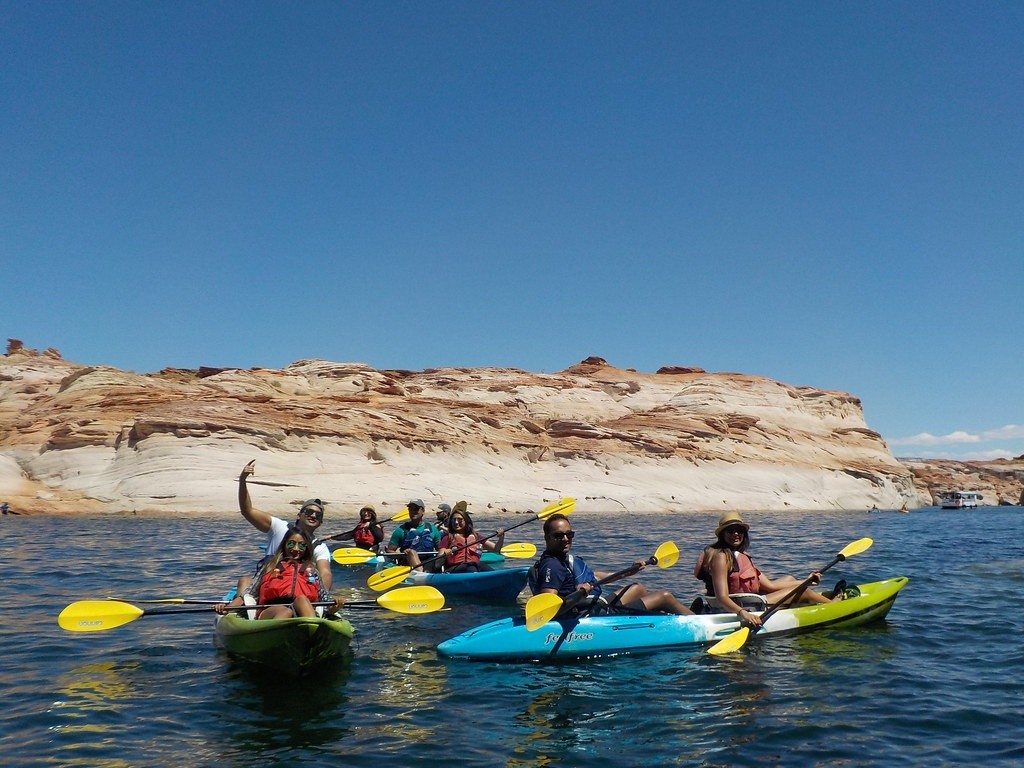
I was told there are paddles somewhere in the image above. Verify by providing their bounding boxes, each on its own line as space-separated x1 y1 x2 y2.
332 543 537 564
706 538 874 655
524 540 680 632
366 497 576 592
106 596 452 613
436 501 468 529
320 507 410 541
57 584 447 631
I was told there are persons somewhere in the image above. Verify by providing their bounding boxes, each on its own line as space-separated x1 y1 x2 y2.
902 505 908 511
703 512 845 627
237 460 332 596
211 528 345 620
872 504 878 510
321 504 384 552
386 498 505 574
537 514 695 617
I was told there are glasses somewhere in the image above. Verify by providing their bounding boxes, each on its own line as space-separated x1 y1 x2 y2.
725 526 746 534
302 508 324 519
548 531 575 539
450 518 465 523
286 541 307 551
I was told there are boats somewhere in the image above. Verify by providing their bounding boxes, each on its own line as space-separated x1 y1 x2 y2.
328 547 532 597
434 576 911 661
899 510 909 514
941 491 978 509
866 509 881 512
212 584 355 666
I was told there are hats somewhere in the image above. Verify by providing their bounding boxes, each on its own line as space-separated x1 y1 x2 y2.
301 498 324 517
432 503 451 514
407 498 425 510
360 504 376 517
715 512 750 536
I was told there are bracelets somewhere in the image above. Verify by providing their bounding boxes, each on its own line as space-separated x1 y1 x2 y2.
736 608 745 615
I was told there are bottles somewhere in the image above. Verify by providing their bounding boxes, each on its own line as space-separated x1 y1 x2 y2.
306 567 315 585
272 568 280 578
